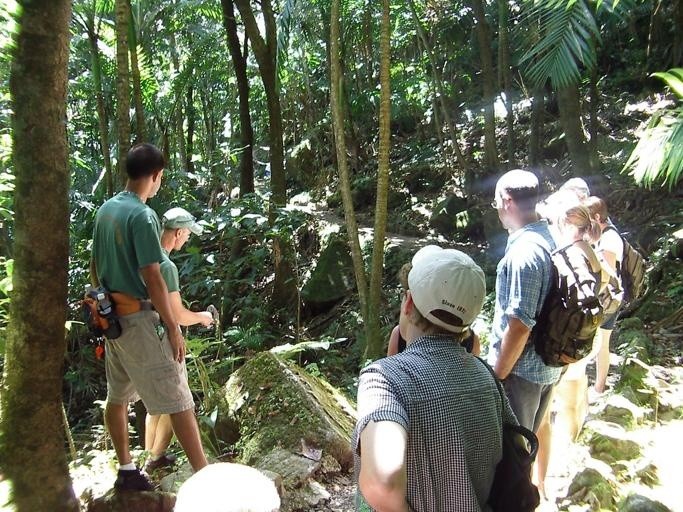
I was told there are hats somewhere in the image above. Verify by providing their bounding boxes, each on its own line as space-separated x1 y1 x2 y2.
162 208 204 235
408 245 487 334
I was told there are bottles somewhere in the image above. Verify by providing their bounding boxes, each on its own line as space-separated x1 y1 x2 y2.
96 293 121 341
83 283 99 303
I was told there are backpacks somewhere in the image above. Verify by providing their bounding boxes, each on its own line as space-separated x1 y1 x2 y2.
474 355 540 512
505 230 602 367
600 228 647 325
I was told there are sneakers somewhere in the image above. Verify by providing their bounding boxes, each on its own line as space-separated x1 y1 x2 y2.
145 453 176 469
115 469 155 491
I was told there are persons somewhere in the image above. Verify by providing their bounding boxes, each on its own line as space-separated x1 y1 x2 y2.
562 178 610 416
544 198 600 444
173 462 281 512
588 197 623 397
89 143 208 491
349 243 529 512
489 167 562 502
388 324 480 357
142 207 212 474
